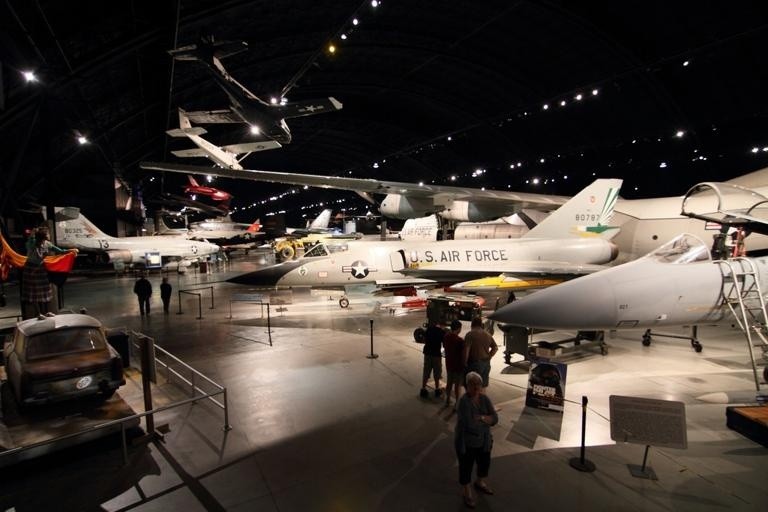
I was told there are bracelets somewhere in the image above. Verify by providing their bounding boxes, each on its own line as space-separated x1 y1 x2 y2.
480 415 484 420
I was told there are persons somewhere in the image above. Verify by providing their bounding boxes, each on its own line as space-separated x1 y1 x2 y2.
419 318 445 399
442 320 465 409
134 274 152 317
19 228 80 320
454 371 499 509
160 278 172 314
461 316 498 393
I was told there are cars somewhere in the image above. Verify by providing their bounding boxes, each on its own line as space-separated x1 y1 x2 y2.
1 311 126 411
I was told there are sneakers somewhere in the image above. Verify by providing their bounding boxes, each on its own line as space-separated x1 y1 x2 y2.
476 480 493 494
419 388 459 413
463 491 475 507
